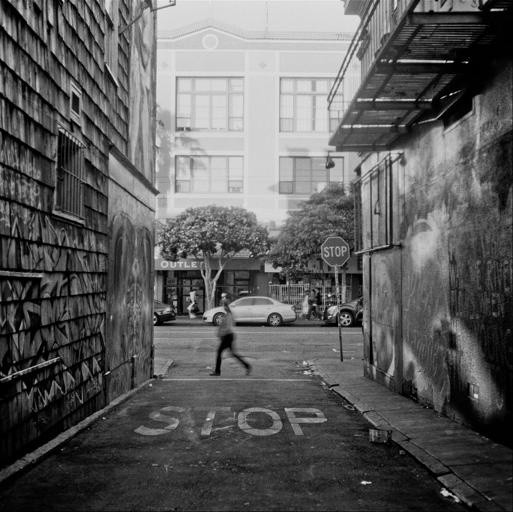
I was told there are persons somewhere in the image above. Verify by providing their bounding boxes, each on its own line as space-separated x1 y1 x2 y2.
314 288 323 321
305 289 324 320
207 297 253 377
300 290 310 321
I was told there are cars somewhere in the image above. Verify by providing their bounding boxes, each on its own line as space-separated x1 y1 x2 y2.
319 294 363 327
153 299 176 325
202 296 298 327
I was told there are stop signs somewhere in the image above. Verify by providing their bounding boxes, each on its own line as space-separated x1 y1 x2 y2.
321 236 350 268
